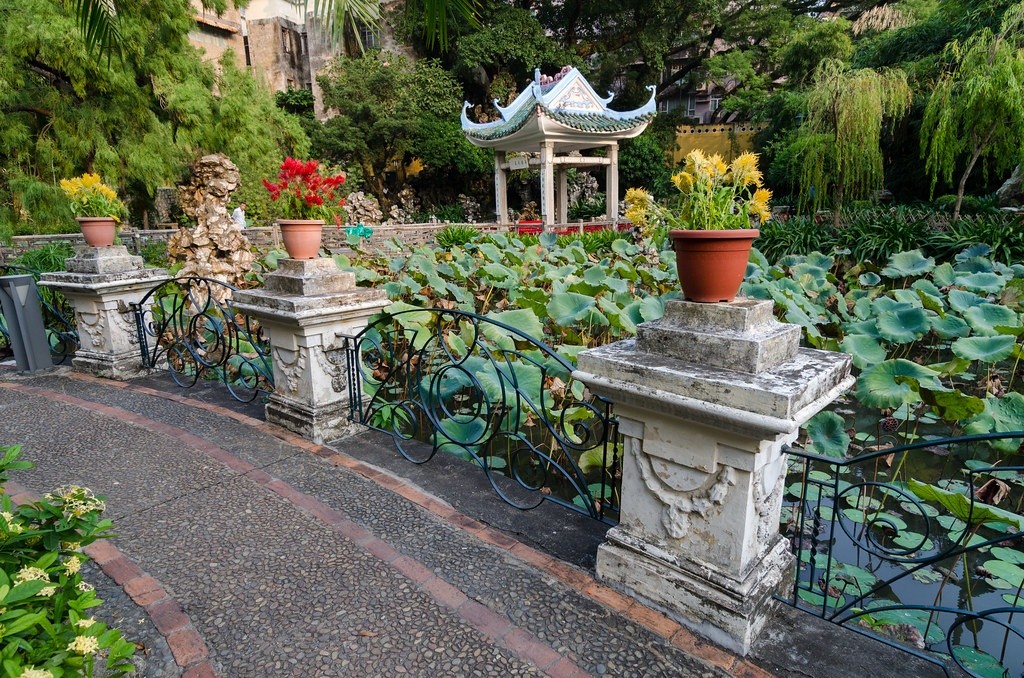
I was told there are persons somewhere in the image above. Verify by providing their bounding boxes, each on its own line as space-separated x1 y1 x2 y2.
231 202 246 229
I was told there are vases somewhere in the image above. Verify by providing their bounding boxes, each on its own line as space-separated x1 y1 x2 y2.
667 229 761 303
276 219 327 261
73 217 117 247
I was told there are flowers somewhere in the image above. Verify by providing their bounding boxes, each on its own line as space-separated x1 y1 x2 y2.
623 147 771 244
262 157 346 230
60 173 125 226
